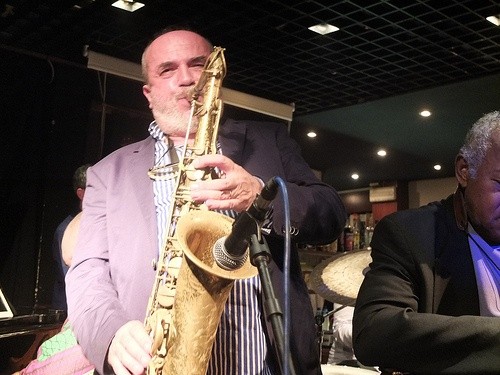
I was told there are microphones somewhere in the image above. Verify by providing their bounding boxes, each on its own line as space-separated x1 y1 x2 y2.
213 176 280 270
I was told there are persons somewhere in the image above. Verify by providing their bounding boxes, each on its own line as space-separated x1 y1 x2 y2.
60 29 347 375
352 111 500 375
53 162 90 320
327 303 381 373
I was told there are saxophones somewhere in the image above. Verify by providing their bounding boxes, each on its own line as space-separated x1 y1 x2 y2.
142 46 259 375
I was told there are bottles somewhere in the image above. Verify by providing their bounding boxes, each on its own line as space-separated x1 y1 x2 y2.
351 216 360 250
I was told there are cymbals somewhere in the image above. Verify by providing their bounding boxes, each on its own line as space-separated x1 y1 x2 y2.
310 247 373 307
